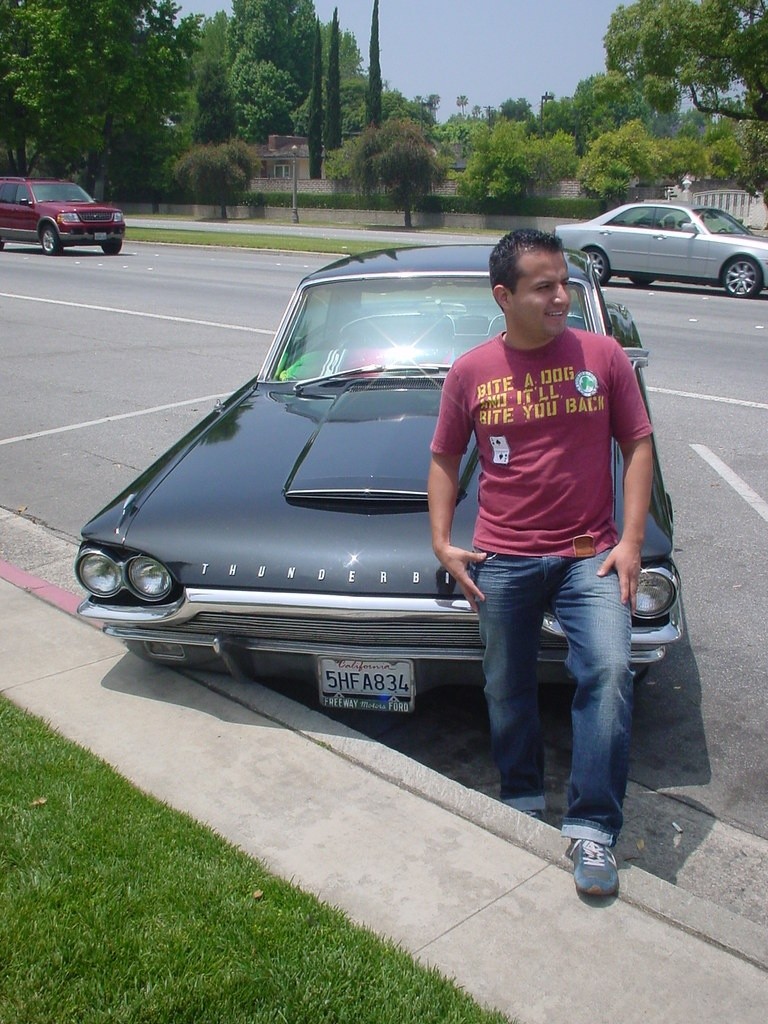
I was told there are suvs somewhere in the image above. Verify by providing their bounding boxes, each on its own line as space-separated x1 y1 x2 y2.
0 177 126 256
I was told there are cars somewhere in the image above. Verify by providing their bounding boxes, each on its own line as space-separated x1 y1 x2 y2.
74 242 686 721
552 199 768 300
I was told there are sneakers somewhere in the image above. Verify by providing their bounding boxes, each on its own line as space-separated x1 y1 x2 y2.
521 810 541 820
569 838 618 895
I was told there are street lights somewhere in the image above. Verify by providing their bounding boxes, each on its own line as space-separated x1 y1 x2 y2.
289 144 300 223
540 91 555 139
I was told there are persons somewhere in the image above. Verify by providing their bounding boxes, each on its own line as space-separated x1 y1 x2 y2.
664 217 676 230
427 228 655 896
